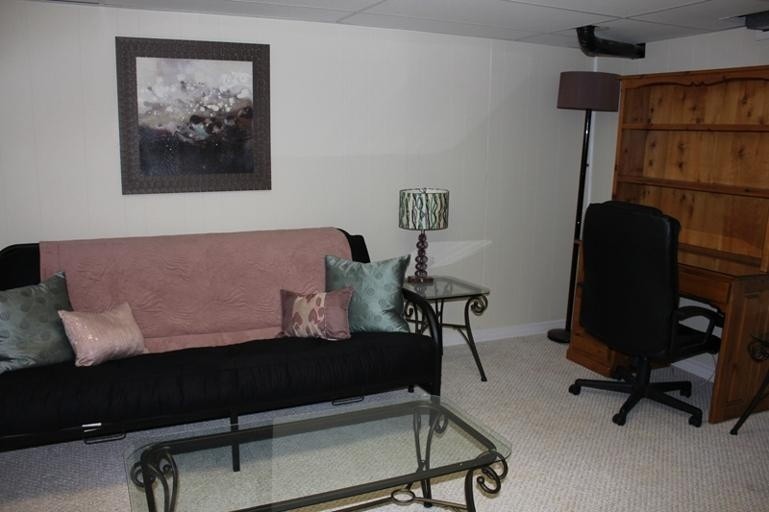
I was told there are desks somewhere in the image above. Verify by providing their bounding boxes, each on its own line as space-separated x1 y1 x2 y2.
566 236 768 422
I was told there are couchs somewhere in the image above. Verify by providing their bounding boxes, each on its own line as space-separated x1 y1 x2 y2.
0 231 443 471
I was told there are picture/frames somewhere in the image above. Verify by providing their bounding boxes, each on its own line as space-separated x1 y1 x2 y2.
114 34 271 193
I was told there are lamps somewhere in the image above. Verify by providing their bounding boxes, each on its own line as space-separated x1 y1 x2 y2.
397 186 450 283
548 71 623 345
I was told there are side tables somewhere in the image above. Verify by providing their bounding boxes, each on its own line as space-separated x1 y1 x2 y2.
404 276 491 382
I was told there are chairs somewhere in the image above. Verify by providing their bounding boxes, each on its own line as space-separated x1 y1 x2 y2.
566 197 725 428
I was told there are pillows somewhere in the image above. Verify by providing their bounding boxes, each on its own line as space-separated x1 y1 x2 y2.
270 255 414 341
0 273 150 374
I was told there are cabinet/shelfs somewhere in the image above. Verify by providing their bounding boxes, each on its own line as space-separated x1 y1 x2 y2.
610 64 769 272
